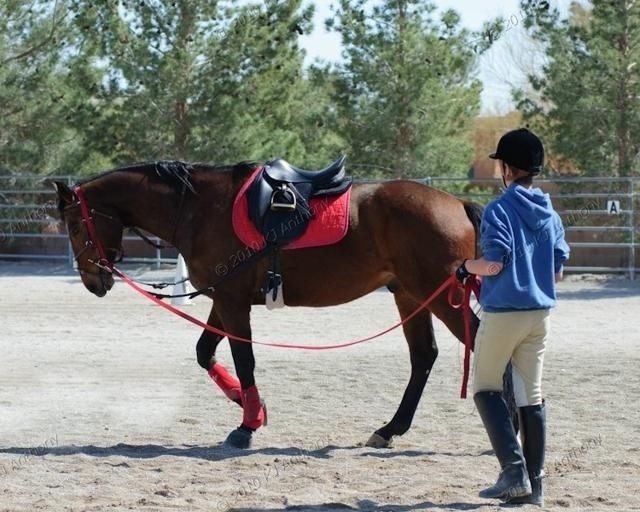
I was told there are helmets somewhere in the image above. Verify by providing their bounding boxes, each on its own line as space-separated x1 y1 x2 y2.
490 128 544 172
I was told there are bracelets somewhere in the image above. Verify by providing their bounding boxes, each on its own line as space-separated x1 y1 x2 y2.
462 259 472 275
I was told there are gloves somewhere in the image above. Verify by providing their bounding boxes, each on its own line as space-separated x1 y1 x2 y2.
455 259 471 285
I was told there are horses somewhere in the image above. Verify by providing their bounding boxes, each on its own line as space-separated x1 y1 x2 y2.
50 159 488 449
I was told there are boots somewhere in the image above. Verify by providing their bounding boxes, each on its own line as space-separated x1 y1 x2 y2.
474 390 546 506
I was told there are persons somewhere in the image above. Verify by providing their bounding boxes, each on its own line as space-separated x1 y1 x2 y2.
452 129 571 505
